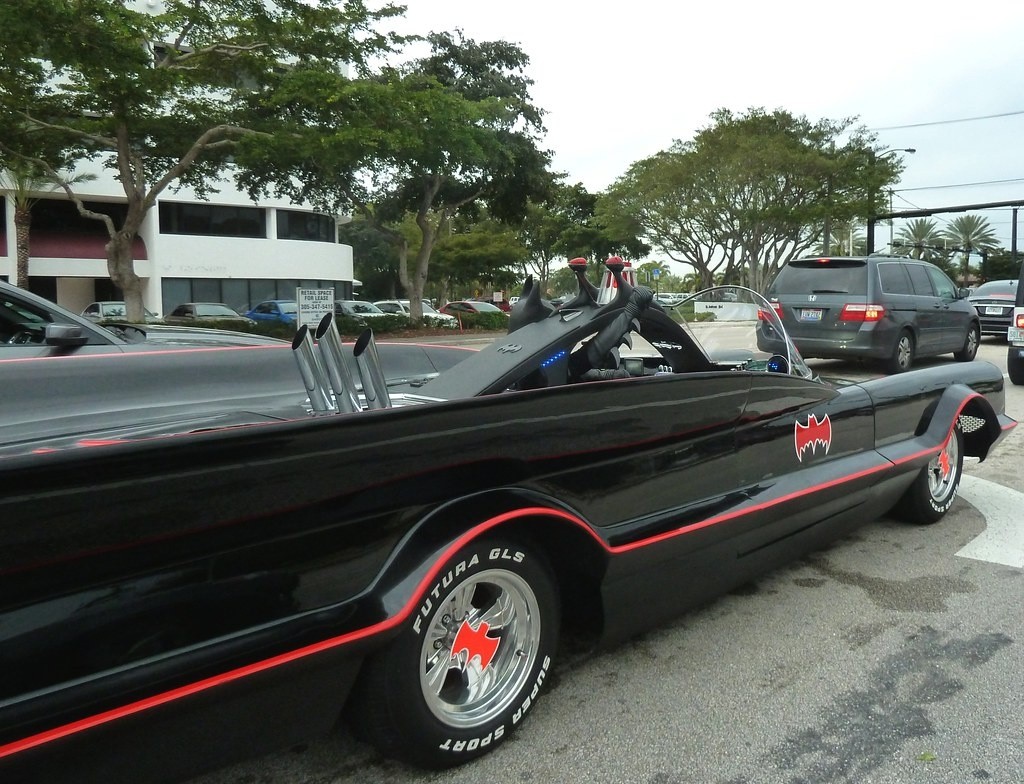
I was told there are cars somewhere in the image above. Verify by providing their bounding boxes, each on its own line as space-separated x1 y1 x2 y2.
1006 258 1024 386
0 277 297 360
969 279 1019 336
244 299 297 327
508 296 522 307
484 297 512 312
436 301 503 319
162 309 258 328
335 300 387 326
373 297 459 329
80 301 169 325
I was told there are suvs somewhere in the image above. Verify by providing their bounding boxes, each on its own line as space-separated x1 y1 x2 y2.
756 253 980 375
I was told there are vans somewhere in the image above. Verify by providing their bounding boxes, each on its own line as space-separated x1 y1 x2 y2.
653 292 737 305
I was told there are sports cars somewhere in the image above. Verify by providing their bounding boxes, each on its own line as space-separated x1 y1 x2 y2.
0 257 1018 783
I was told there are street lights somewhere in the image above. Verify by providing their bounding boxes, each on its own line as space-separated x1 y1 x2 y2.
867 147 916 255
